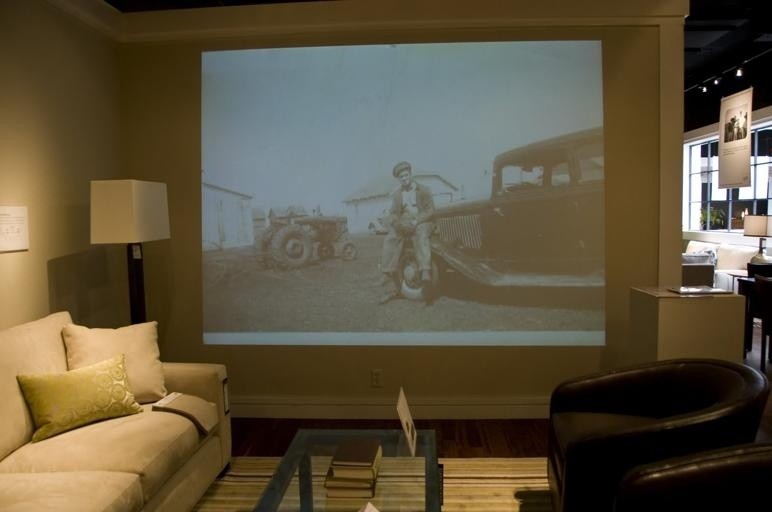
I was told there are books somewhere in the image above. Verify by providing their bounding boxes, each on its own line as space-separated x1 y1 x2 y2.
323 439 383 502
665 285 735 295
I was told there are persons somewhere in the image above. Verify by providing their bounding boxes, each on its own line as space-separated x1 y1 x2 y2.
378 162 437 304
724 110 747 143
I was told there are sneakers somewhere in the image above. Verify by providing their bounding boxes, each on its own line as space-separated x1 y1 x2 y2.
379 289 398 304
421 269 431 282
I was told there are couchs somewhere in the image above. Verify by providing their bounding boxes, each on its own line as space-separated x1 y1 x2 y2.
0 310 232 512
683 240 771 294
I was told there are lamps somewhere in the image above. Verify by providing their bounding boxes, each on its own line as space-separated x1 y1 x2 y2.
743 213 771 264
90 178 172 327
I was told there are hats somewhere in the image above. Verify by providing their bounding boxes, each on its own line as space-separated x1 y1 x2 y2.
393 162 412 177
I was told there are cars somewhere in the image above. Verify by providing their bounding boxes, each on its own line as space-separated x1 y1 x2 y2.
388 124 606 305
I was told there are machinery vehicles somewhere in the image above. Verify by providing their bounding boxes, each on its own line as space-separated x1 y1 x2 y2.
253 204 357 274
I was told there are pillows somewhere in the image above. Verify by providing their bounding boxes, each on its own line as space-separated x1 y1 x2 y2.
685 241 759 271
15 321 167 443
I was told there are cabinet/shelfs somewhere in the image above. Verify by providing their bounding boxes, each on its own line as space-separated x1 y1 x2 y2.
629 286 745 363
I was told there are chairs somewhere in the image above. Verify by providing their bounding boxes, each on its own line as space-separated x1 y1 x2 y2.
547 358 772 511
747 263 771 373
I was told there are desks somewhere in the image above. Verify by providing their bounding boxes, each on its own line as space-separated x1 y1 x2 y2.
738 277 771 362
